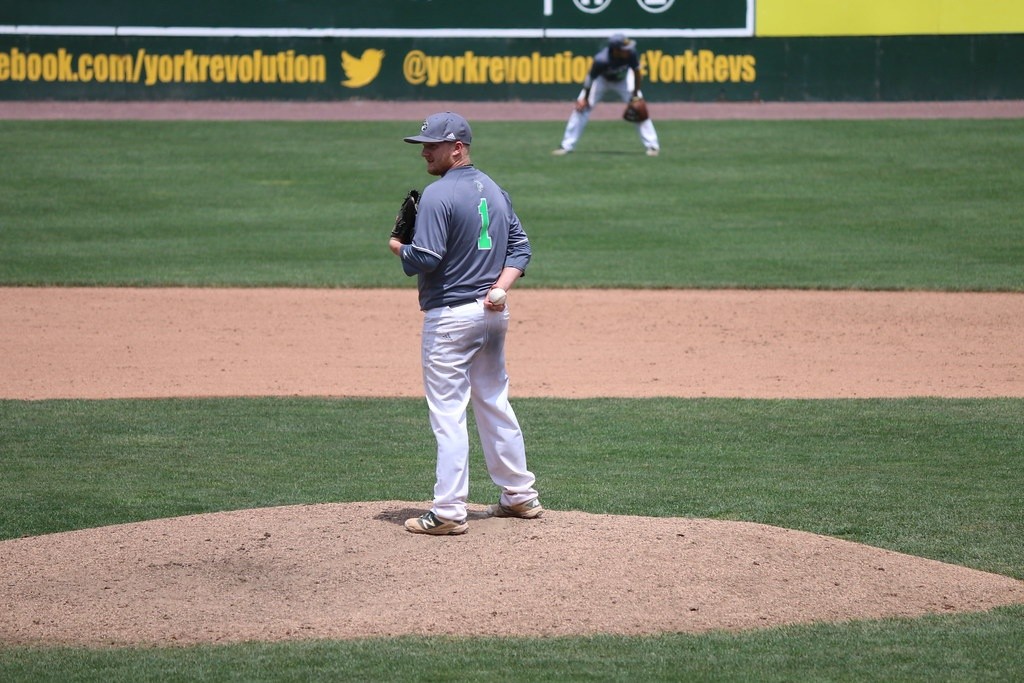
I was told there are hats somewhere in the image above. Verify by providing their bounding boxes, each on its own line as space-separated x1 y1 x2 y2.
609 33 637 51
403 111 472 145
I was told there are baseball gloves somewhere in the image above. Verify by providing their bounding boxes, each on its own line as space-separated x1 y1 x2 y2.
390 189 421 245
623 92 650 123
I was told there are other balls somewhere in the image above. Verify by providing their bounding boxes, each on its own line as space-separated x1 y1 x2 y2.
488 287 507 305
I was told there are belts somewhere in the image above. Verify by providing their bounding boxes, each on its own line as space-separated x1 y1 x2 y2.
449 299 477 308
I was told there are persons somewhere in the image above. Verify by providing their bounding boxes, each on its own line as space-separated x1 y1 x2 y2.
551 33 660 157
389 111 544 535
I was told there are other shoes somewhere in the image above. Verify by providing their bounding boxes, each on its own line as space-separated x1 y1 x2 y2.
552 145 571 155
646 146 659 156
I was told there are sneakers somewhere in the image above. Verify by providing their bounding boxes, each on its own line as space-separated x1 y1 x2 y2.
494 497 545 518
405 512 469 535
